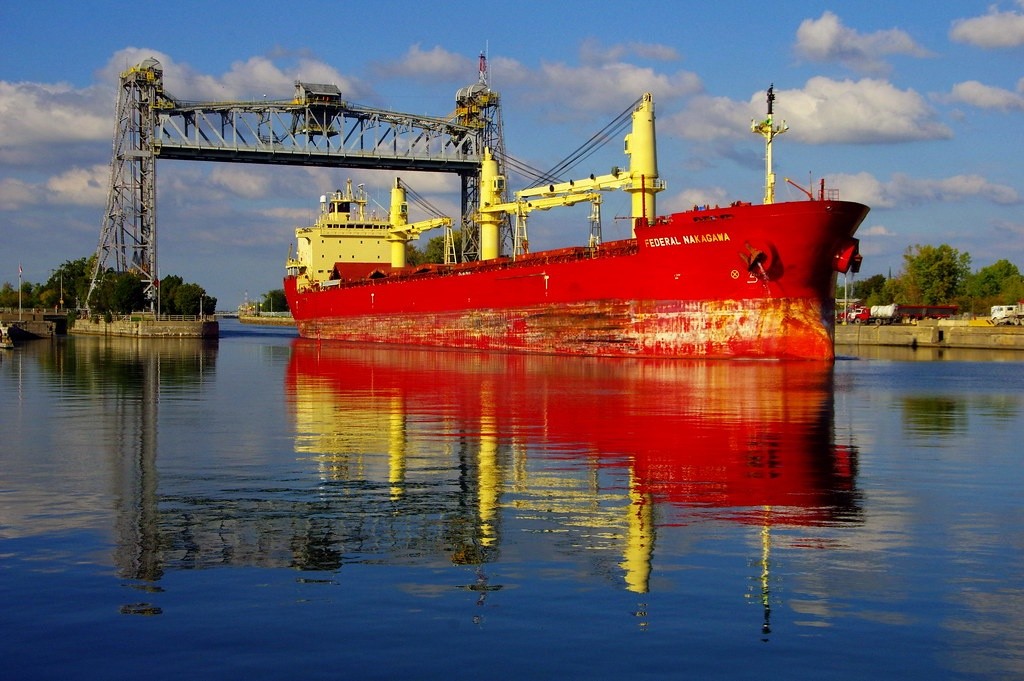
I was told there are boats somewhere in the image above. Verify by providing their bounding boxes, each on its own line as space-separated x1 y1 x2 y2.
282 82 871 363
283 335 863 634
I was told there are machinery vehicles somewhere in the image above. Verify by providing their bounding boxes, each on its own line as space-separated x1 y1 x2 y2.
849 305 898 325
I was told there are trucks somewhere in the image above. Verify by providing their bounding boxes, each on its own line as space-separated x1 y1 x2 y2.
991 305 1024 326
